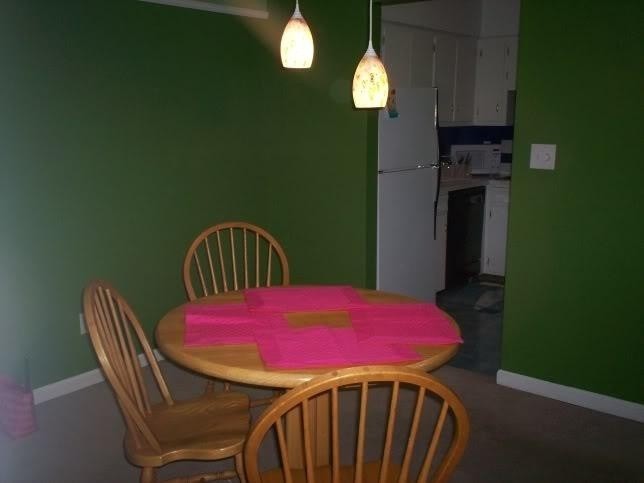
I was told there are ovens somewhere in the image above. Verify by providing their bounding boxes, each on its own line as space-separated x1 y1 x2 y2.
446 185 487 288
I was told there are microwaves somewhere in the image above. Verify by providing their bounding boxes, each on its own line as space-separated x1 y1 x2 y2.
449 142 504 177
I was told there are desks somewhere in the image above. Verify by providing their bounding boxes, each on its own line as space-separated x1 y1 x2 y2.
154 284 463 468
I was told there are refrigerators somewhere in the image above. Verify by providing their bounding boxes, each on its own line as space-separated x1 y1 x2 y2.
374 84 438 304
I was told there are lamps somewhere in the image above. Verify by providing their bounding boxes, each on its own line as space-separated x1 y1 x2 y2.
278 0 316 70
352 0 390 110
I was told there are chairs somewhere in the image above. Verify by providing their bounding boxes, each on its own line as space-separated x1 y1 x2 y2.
183 224 288 410
242 363 471 483
83 277 252 481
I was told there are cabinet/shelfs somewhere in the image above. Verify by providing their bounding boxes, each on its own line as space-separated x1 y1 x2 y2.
478 180 511 276
504 35 519 91
436 191 449 293
380 22 435 89
433 33 476 127
476 37 513 127
